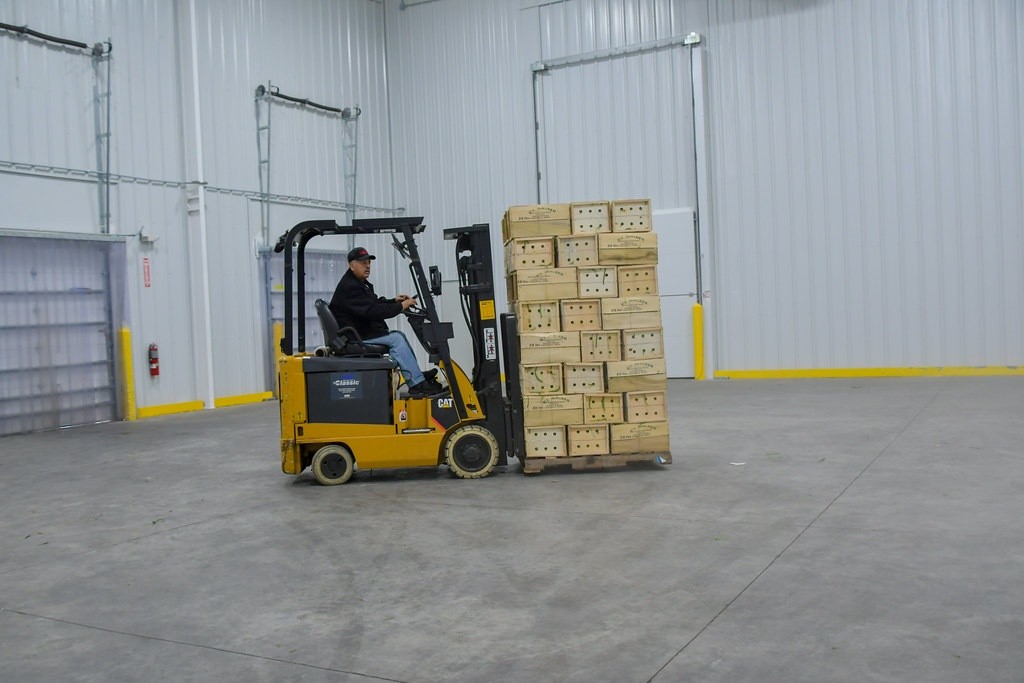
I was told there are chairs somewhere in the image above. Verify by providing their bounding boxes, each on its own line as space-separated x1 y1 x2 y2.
315 293 393 358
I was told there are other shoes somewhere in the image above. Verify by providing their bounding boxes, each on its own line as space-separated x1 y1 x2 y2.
421 368 438 382
408 381 442 395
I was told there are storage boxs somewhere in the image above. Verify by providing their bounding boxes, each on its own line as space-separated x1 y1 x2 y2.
500 197 681 477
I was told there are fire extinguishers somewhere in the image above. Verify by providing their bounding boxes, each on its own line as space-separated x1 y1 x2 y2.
149 343 159 376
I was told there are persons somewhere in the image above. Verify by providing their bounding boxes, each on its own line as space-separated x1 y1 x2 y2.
329 247 442 394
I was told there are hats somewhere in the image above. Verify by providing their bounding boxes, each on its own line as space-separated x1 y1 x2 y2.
348 247 376 263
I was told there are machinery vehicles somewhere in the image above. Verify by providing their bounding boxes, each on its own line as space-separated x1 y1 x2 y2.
274 216 673 487
